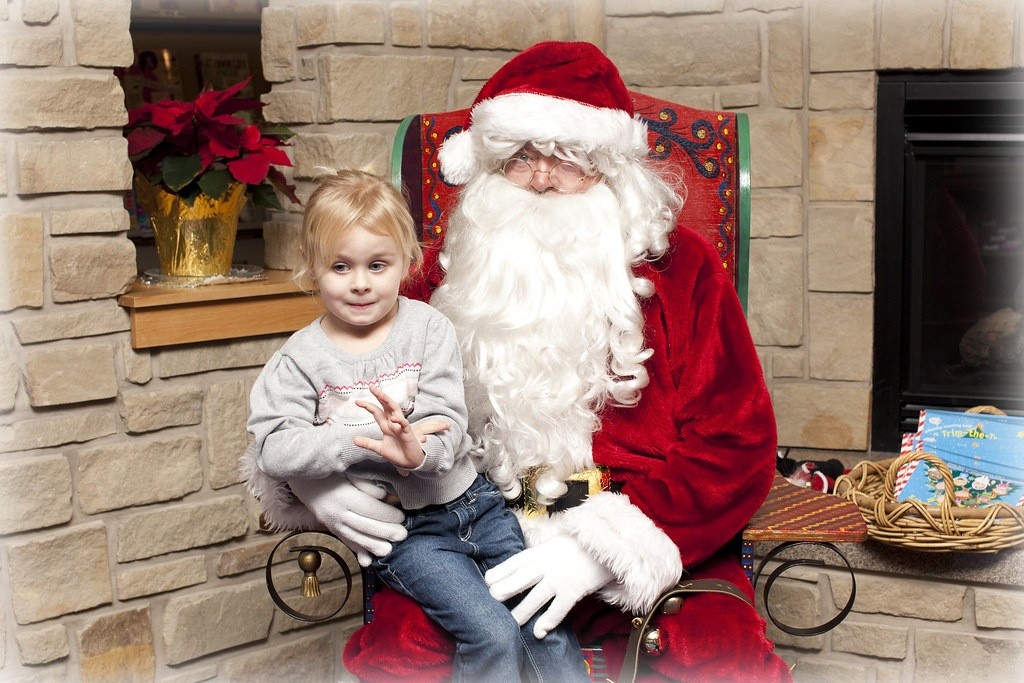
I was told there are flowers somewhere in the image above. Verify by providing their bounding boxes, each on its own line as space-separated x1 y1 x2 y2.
127 73 309 215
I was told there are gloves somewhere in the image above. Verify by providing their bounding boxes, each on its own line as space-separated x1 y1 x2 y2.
481 529 617 642
285 477 411 569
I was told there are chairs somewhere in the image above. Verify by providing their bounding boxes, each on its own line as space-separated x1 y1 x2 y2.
265 72 871 680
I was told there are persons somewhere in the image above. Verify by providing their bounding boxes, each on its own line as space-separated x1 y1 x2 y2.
339 36 785 683
237 169 592 682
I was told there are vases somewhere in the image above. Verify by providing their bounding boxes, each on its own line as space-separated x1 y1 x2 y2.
136 163 253 278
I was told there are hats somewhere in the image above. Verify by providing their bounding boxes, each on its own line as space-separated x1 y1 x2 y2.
469 42 644 163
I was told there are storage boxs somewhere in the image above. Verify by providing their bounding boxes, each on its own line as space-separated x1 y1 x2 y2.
827 403 1022 556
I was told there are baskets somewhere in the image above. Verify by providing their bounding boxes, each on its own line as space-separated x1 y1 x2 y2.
833 404 1023 554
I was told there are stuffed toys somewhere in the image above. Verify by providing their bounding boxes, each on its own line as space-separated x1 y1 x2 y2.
775 446 852 494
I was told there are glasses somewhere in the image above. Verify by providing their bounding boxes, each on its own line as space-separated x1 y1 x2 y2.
499 157 589 195
